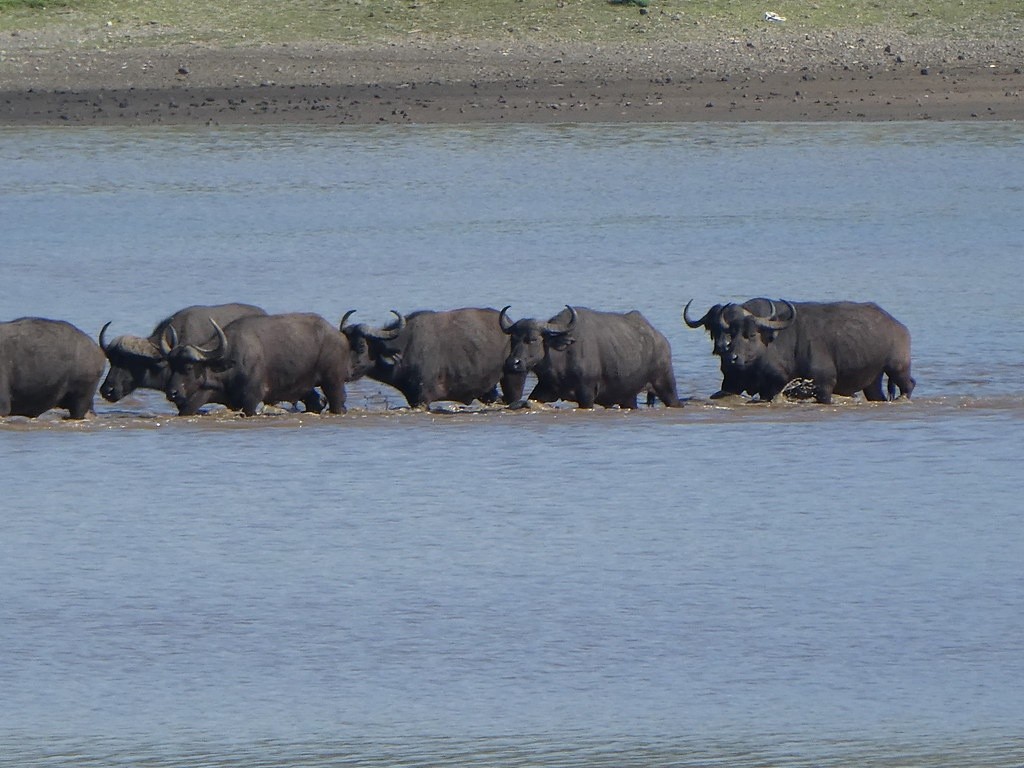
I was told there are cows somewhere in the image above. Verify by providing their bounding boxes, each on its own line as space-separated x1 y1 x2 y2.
0 302 684 420
683 297 916 404
